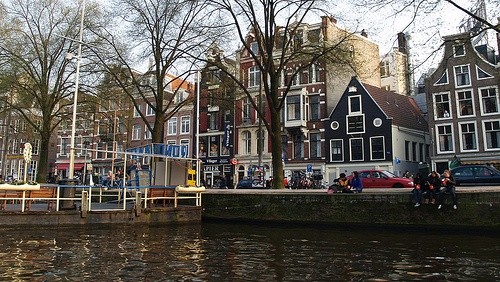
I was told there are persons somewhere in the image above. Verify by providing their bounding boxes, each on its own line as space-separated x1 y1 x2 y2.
438 170 457 210
331 173 348 193
403 170 409 178
411 173 426 207
284 177 288 185
269 176 273 187
425 171 441 204
346 171 363 193
116 170 123 178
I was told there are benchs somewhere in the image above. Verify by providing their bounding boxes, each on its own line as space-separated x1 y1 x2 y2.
143 188 176 208
16 189 55 211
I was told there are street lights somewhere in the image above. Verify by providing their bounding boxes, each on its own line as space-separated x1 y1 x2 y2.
63 0 86 180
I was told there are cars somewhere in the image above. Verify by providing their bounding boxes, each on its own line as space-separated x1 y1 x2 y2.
440 164 500 187
343 169 414 188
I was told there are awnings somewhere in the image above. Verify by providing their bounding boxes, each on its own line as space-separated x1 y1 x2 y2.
57 164 84 170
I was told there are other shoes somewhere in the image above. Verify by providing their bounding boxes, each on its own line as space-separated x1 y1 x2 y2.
438 205 441 209
431 199 435 204
414 203 420 207
454 205 457 209
425 199 429 204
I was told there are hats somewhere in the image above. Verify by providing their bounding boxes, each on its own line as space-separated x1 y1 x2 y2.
340 173 346 178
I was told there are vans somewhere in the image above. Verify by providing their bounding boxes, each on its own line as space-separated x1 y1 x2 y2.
187 169 196 187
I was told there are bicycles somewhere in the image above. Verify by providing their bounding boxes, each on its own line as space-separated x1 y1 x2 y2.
252 169 329 190
